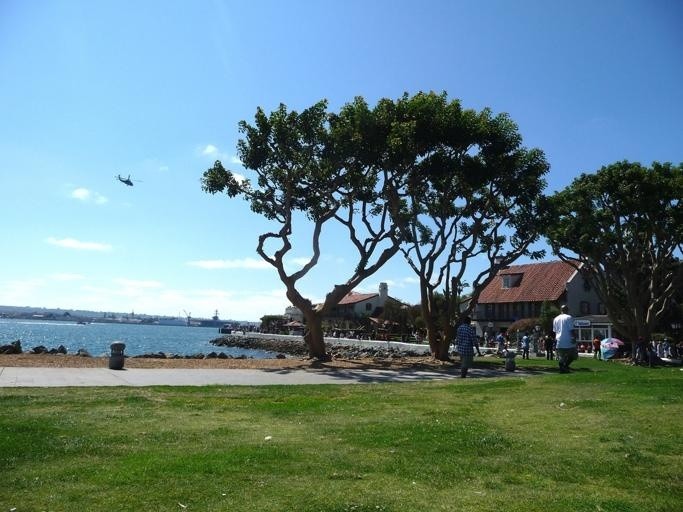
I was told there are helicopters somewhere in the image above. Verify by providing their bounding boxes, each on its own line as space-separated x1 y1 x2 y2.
114 173 144 186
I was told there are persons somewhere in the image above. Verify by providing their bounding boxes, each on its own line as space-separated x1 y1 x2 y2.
496 333 683 360
457 316 481 378
553 305 577 373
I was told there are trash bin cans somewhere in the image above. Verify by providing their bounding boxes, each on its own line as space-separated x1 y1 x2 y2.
109 342 126 370
